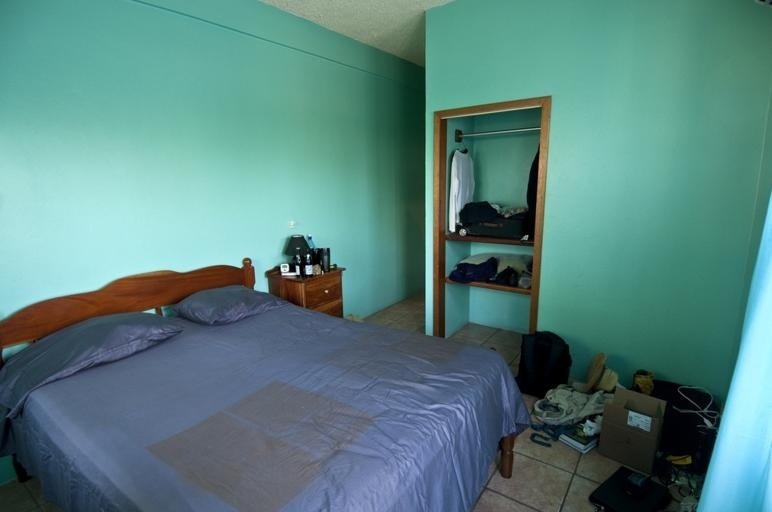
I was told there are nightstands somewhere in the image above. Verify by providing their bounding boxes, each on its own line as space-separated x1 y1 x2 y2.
265 266 346 317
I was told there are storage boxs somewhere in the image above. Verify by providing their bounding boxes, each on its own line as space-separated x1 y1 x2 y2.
598 387 667 474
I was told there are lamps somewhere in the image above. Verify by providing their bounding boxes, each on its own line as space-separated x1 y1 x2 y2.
285 235 311 276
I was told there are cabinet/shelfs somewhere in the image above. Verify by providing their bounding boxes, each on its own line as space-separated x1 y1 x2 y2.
433 96 552 338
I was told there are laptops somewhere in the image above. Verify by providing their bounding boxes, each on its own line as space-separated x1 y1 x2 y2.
589 467 673 512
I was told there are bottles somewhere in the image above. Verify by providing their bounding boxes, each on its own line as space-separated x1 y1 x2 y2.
307 233 314 251
293 247 313 278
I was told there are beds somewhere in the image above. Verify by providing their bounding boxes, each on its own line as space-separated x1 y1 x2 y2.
0 257 531 512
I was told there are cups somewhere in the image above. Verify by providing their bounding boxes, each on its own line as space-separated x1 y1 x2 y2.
321 248 330 271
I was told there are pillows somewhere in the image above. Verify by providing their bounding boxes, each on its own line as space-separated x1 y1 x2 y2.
171 284 278 324
0 312 186 420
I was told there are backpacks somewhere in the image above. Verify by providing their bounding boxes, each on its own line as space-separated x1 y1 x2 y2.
515 329 572 399
